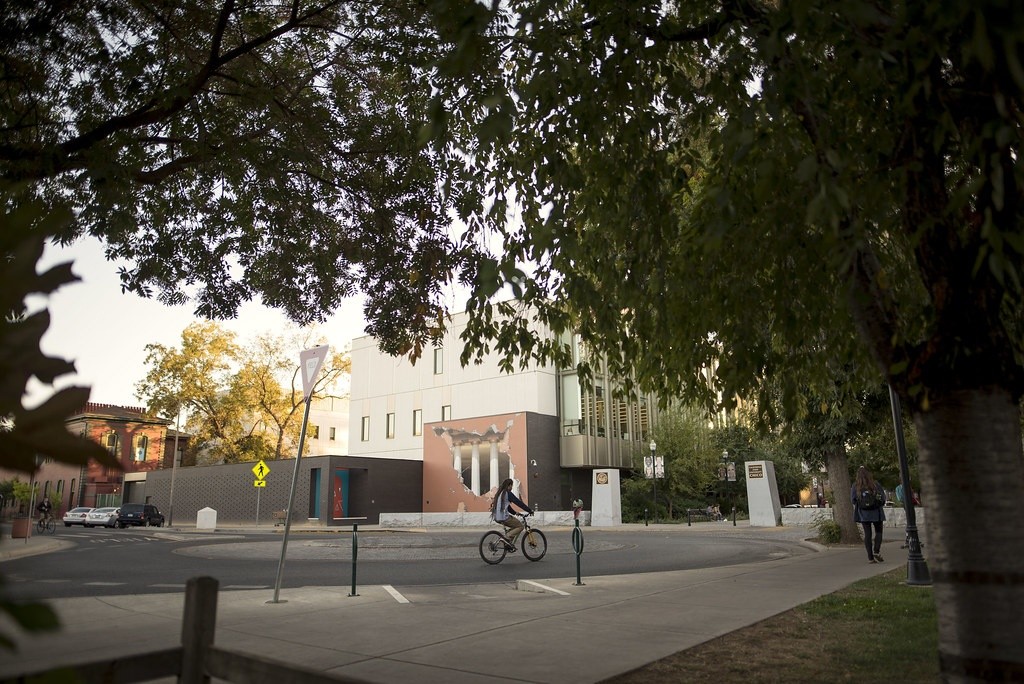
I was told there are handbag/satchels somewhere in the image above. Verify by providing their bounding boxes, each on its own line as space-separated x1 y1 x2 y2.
860 489 884 510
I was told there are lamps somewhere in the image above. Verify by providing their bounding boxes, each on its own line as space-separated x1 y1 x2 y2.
645 508 648 512
687 509 691 511
731 507 735 510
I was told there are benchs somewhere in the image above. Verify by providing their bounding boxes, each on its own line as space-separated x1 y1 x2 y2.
688 508 711 523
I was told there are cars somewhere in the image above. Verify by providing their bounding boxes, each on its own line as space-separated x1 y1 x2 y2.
86 507 122 529
62 507 95 527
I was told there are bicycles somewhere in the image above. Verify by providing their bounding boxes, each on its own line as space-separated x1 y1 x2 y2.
479 510 548 564
36 510 56 535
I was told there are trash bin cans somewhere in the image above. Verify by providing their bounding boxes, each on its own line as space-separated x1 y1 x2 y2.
12 517 33 538
196 507 217 529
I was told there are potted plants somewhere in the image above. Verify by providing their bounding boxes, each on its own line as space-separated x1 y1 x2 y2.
10 480 40 538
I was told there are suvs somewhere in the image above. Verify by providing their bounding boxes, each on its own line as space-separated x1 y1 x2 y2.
117 503 165 528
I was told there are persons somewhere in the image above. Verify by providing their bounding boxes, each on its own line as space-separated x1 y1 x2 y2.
851 467 887 563
490 478 533 548
896 484 917 515
706 503 723 522
573 496 583 520
37 497 52 529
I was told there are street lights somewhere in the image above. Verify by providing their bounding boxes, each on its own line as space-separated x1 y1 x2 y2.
722 449 729 517
866 223 933 586
649 438 659 524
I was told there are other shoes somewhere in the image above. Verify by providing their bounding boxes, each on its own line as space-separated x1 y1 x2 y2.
868 559 877 563
872 551 884 562
499 535 512 547
